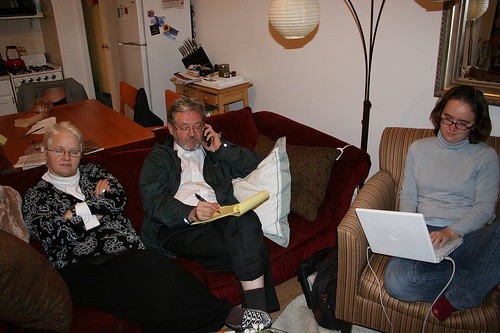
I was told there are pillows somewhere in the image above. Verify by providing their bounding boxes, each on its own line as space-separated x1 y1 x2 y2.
0 185 30 244
0 230 72 332
230 136 291 248
254 133 341 222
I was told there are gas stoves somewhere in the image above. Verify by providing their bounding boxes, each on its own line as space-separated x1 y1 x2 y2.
2 53 62 78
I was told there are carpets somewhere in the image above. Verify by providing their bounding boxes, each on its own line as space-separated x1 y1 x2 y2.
270 294 385 333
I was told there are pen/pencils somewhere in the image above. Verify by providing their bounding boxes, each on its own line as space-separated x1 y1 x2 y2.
31 126 44 133
194 193 222 215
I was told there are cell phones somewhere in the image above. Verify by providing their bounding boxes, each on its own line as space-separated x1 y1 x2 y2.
203 123 212 147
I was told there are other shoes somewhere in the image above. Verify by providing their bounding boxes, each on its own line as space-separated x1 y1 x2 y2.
432 295 456 322
265 285 281 313
223 308 272 331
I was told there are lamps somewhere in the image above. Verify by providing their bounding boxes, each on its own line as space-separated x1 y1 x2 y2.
467 0 490 21
269 0 385 189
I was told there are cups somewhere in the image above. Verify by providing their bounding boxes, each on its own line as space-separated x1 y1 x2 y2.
218 64 230 78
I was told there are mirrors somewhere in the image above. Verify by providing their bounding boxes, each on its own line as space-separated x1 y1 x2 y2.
434 0 500 105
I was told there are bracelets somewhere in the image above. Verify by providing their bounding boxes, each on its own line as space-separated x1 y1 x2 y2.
184 218 190 224
70 206 77 217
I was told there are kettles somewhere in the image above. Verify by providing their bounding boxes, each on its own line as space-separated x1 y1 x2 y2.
0 45 25 71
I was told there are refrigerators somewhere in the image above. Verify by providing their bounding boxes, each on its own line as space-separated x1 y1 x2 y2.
114 0 196 126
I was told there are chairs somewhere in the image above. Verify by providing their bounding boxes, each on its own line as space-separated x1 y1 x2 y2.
165 89 182 126
17 78 89 113
120 81 139 117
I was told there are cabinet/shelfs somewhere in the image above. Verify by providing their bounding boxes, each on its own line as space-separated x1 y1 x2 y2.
0 79 17 116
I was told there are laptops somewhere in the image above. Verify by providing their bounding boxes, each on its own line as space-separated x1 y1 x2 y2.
355 208 463 264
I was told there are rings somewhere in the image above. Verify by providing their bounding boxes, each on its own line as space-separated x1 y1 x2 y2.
102 189 106 192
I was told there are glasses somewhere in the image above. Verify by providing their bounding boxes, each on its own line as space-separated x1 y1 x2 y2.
168 120 206 134
439 111 475 130
46 147 81 157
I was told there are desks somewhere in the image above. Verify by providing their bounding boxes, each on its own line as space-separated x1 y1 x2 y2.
176 82 253 114
0 99 157 173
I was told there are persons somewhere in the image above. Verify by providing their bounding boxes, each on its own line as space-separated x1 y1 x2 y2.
138 97 288 333
384 85 500 322
21 121 272 333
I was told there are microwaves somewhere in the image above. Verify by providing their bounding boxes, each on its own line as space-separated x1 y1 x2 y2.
0 0 44 20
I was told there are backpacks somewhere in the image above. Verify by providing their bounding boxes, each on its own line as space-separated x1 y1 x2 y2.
298 247 346 330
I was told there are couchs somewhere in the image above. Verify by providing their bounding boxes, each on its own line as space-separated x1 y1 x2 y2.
336 126 500 333
0 112 372 333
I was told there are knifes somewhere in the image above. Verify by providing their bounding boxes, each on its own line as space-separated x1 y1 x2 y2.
178 37 199 57
25 126 44 138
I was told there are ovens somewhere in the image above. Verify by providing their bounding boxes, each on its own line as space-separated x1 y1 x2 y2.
12 72 64 104
